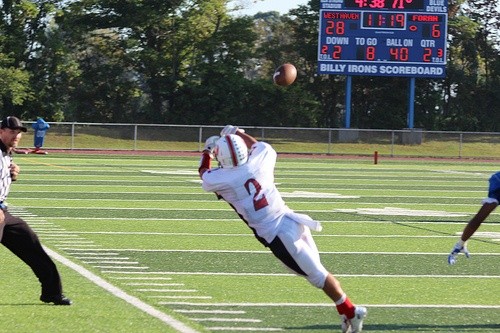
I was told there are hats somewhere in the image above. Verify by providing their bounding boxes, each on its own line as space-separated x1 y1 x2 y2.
1 116 27 132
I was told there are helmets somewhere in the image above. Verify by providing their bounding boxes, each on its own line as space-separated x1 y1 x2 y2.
215 133 248 169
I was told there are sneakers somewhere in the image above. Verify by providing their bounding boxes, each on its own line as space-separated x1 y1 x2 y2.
350 306 367 333
340 313 349 333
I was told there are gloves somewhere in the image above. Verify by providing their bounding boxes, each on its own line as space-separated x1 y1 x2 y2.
202 135 220 159
219 125 239 136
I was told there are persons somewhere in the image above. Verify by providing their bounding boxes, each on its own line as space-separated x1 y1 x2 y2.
198 125 367 332
446 169 500 264
0 115 73 306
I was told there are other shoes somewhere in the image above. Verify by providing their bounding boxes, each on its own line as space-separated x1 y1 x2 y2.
40 294 72 305
448 242 470 266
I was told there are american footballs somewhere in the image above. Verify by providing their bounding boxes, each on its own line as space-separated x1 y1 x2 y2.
273 62 298 88
